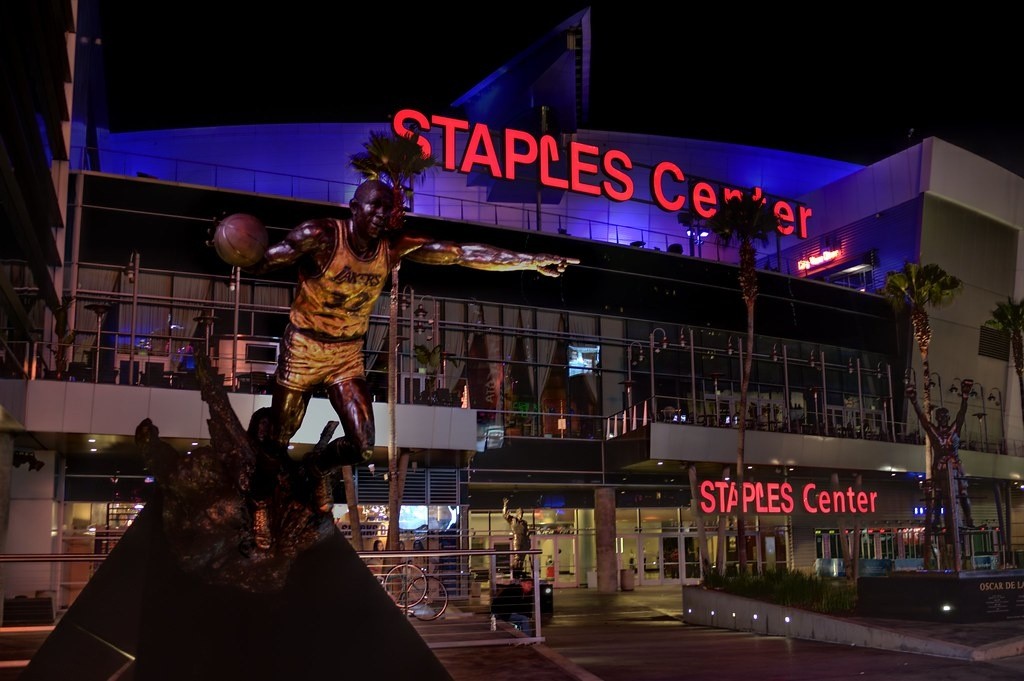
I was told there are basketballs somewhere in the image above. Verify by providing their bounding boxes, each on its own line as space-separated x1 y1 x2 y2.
214 213 268 268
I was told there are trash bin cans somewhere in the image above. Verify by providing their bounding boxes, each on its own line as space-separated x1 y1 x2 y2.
620 568 635 591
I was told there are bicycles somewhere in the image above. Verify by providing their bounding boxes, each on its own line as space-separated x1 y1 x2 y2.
375 564 448 622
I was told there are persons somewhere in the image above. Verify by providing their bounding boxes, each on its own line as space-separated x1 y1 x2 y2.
204 178 582 515
502 495 532 571
628 545 702 579
491 575 536 636
365 538 440 584
906 378 977 533
